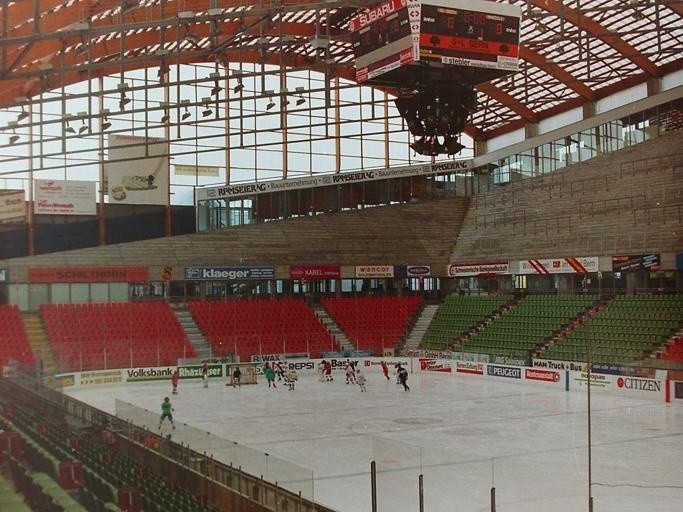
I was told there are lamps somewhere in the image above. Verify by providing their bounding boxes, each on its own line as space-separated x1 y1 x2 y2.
396 74 477 157
6 8 330 144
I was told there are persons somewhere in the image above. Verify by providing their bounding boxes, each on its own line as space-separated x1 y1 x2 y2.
233 367 241 388
381 361 390 381
344 362 355 384
266 365 276 388
288 370 296 391
203 364 208 387
356 369 367 392
172 371 178 392
275 363 285 383
349 361 356 380
396 364 410 391
157 397 176 430
321 360 333 381
393 370 402 384
